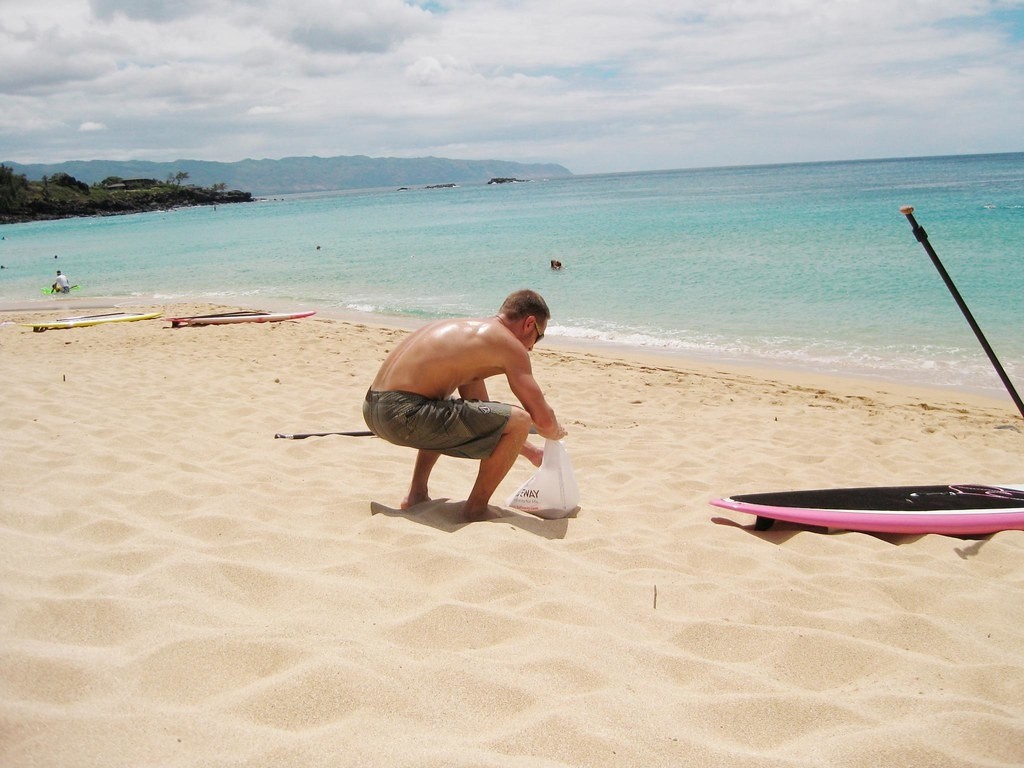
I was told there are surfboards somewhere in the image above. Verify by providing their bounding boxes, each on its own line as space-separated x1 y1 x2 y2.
708 480 1024 538
161 310 316 323
21 312 163 325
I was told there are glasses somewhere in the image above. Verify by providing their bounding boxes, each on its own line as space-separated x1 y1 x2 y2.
534 320 544 344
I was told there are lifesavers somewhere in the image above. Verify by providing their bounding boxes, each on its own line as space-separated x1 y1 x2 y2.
41 284 80 296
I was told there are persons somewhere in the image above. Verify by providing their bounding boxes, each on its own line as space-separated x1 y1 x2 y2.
362 289 568 522
54 271 70 294
551 257 562 270
